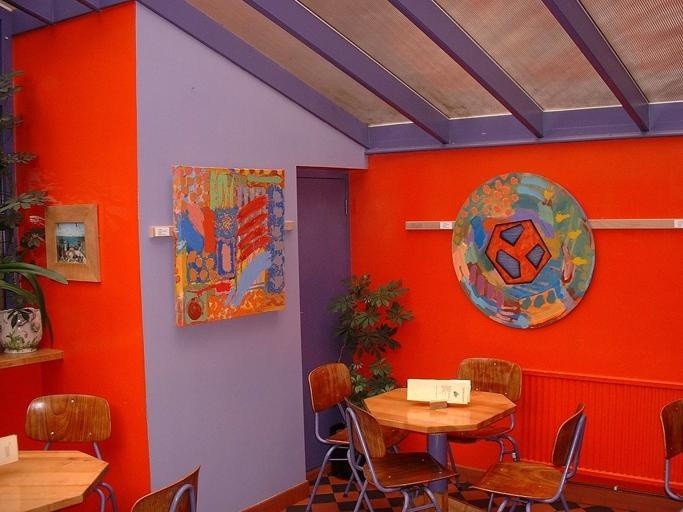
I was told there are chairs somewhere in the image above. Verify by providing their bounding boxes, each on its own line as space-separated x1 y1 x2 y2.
301 362 406 512
25 393 117 512
650 396 682 510
442 353 521 491
129 462 202 512
466 396 588 512
339 396 458 512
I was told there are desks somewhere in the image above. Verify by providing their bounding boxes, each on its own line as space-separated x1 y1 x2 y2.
361 384 514 512
0 442 107 512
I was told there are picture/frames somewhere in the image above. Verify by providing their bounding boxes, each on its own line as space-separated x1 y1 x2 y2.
42 203 102 283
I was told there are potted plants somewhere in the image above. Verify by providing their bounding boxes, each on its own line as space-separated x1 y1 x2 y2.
326 274 412 477
0 64 55 354
